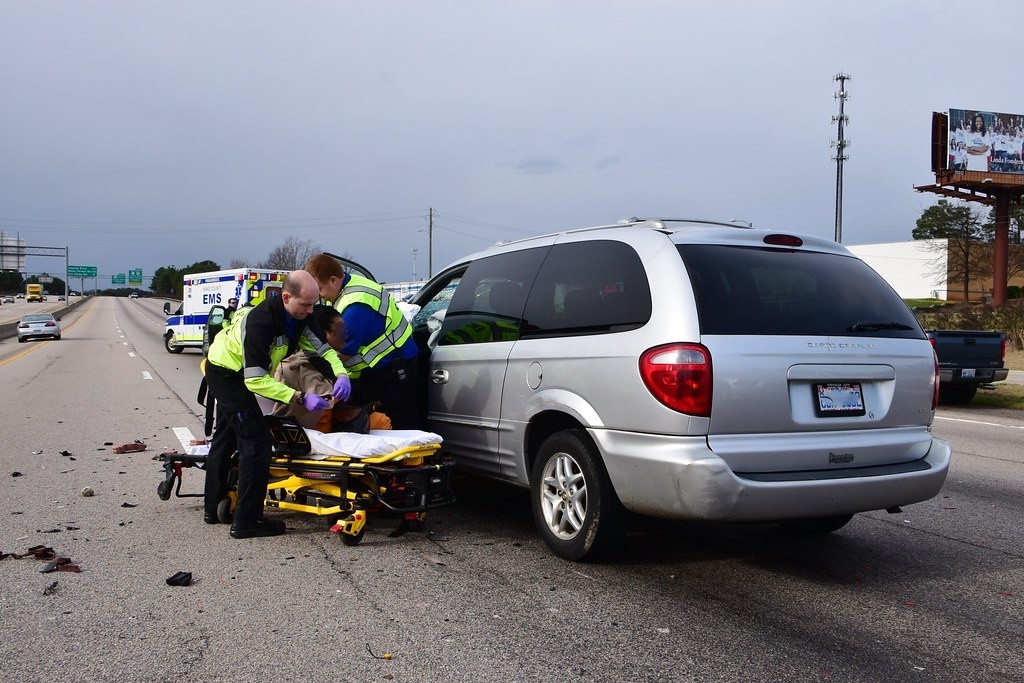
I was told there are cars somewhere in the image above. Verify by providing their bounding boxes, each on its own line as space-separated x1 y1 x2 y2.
17 312 60 343
129 292 139 299
16 292 25 300
403 212 955 562
58 296 66 301
70 292 76 296
3 296 14 303
42 295 48 302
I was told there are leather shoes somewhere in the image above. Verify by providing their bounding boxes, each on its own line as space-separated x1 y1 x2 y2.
204 512 221 524
229 515 286 538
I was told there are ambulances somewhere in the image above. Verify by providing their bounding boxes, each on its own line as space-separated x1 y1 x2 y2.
162 268 299 354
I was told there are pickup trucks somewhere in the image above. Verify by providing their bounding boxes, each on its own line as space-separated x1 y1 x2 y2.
923 329 1009 408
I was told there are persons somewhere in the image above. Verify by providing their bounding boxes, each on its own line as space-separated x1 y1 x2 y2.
949 114 1024 172
304 254 419 430
204 270 351 539
222 298 238 329
254 304 392 434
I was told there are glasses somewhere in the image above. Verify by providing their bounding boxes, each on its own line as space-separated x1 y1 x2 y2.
231 302 237 304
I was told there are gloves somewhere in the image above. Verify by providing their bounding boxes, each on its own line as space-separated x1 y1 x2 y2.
302 392 328 412
332 374 351 402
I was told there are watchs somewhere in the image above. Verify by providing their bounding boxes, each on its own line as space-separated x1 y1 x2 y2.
297 391 306 406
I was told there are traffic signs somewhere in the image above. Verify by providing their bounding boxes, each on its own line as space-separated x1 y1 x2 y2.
112 273 125 285
129 268 142 286
67 265 97 278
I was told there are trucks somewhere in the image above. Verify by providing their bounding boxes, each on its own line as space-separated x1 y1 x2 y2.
26 284 43 303
